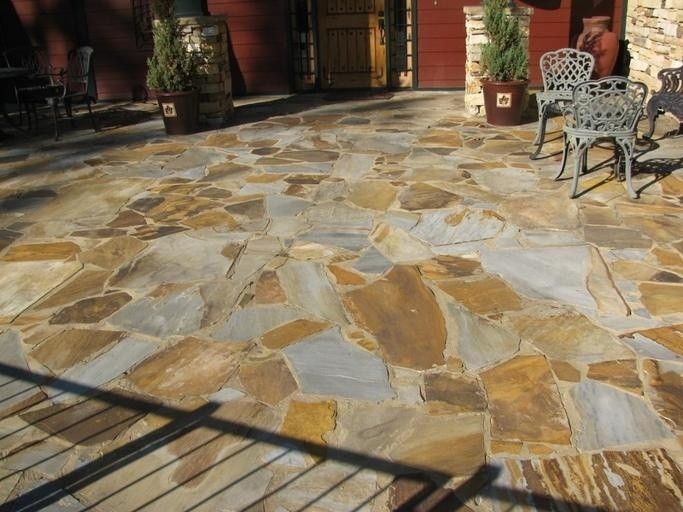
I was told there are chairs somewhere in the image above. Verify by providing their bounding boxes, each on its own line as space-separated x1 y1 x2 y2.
529 47 683 201
0 46 98 141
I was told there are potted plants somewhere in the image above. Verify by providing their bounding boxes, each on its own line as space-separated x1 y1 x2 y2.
477 1 531 125
145 1 208 134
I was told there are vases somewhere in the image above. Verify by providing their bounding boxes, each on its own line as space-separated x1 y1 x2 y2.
573 15 619 80
